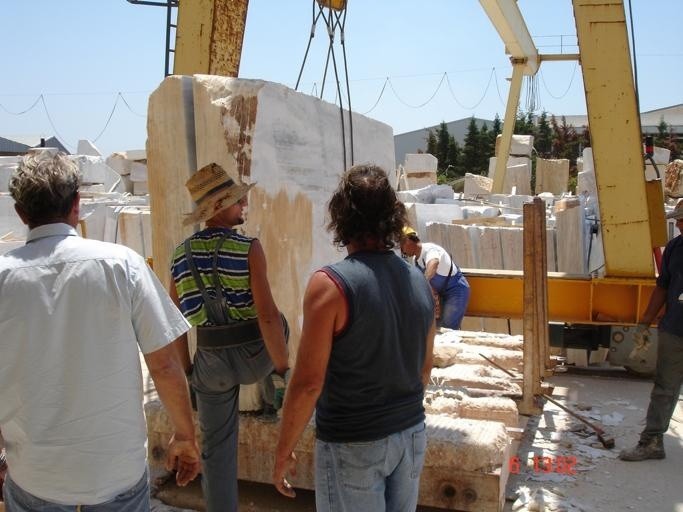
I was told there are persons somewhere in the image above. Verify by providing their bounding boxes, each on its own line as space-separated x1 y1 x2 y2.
398 226 470 332
618 198 683 462
0 149 202 512
165 163 291 512
272 163 437 512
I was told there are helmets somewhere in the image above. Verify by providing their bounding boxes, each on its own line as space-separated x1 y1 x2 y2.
401 225 417 237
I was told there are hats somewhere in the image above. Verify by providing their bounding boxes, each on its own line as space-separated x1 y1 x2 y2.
664 200 683 220
181 162 258 227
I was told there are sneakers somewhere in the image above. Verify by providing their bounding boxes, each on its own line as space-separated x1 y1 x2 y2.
619 433 666 462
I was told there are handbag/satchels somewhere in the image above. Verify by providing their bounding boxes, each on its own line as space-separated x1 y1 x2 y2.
430 288 442 321
